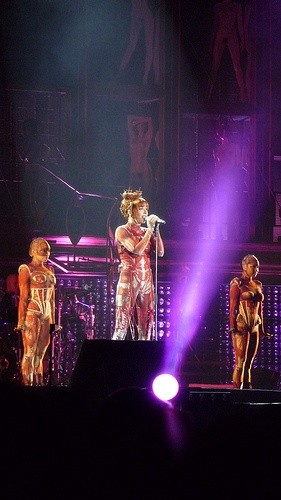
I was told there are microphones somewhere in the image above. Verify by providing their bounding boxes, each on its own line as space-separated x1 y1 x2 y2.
144 216 166 225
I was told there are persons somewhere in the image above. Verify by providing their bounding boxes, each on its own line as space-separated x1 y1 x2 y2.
229 254 272 389
111 188 165 341
16 114 248 227
0 365 281 500
0 273 20 323
14 237 62 387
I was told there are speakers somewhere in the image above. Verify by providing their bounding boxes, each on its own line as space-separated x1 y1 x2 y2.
70 339 165 390
